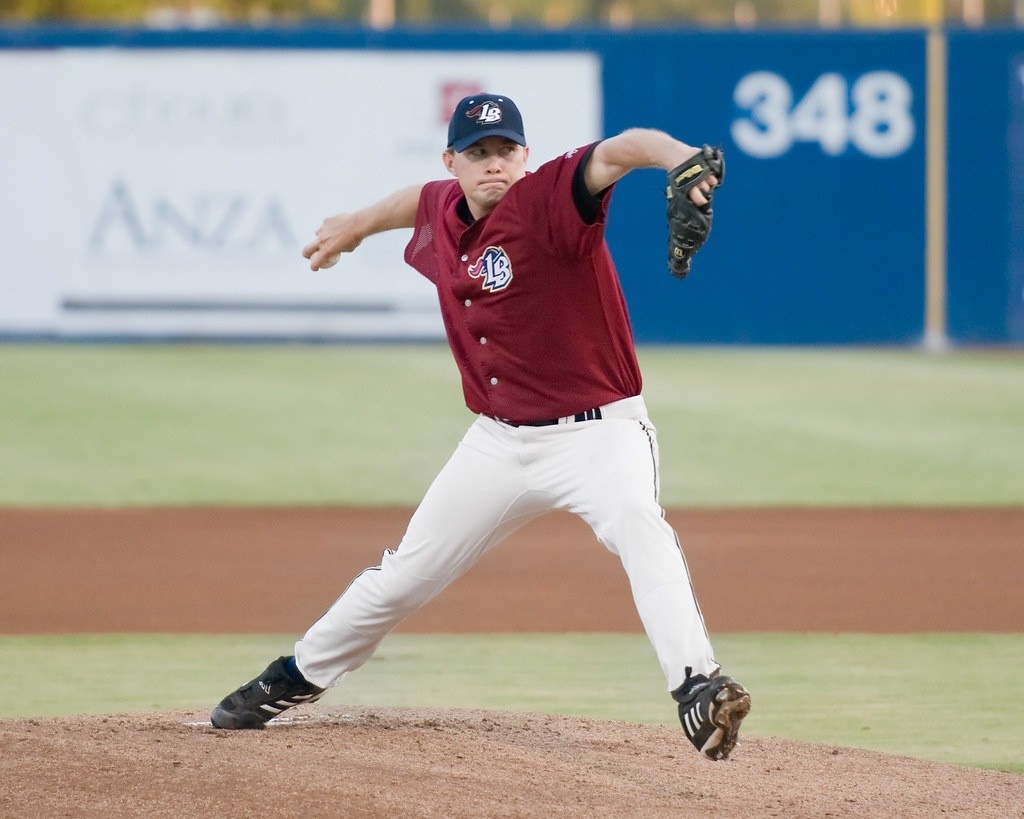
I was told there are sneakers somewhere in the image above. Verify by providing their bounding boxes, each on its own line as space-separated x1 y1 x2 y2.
211 656 329 730
671 666 751 761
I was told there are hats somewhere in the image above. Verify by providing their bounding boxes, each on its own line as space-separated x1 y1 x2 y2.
447 92 526 153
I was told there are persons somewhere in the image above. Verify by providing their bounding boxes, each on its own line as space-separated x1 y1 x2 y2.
212 92 750 762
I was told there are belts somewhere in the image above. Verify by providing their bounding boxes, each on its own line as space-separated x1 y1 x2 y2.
502 408 602 428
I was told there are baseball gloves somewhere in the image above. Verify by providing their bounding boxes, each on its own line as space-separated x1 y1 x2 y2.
664 143 725 279
